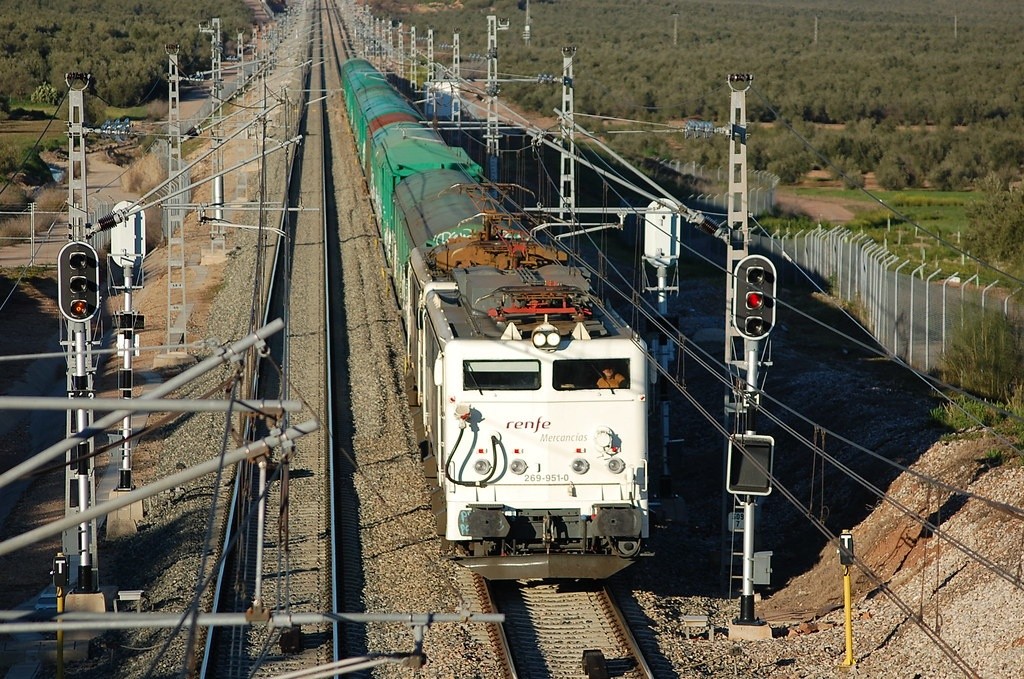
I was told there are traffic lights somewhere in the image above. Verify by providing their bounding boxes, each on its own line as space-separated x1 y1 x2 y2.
734 255 778 339
54 244 100 320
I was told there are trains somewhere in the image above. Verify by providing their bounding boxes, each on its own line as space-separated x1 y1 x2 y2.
340 56 653 583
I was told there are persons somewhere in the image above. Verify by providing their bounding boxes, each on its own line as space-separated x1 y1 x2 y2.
594 360 627 389
